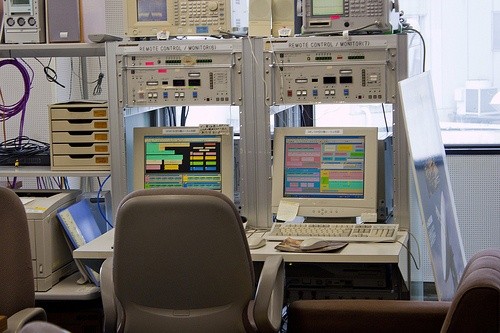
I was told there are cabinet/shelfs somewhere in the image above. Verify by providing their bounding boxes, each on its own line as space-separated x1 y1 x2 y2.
0 43 111 177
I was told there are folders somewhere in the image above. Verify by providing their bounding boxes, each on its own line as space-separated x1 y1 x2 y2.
57 198 102 288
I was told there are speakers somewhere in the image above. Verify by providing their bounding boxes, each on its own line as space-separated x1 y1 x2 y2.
44 0 81 42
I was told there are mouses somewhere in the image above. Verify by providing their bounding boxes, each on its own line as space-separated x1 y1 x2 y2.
300 237 328 249
246 230 266 249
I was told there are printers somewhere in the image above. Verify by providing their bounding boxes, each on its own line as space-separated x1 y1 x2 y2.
8 187 83 292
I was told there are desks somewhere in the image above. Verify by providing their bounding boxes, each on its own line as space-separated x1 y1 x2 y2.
34 270 99 300
72 226 409 262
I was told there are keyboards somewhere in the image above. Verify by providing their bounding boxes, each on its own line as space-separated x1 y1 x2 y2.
266 223 398 243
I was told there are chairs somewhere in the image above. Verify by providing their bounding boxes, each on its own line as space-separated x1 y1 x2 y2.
289 249 500 333
0 187 47 333
100 188 285 333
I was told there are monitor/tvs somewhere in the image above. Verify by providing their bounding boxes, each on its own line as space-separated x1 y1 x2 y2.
270 128 378 222
133 128 235 203
